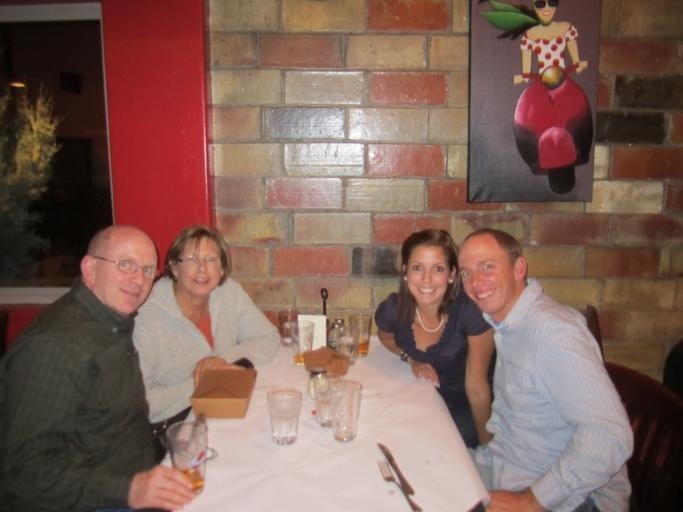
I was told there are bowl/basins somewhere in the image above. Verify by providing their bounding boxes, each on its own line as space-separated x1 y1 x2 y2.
415 307 446 333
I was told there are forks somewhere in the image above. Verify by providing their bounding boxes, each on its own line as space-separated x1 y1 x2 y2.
376 458 423 512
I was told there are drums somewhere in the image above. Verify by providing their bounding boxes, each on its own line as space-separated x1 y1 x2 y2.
376 442 415 496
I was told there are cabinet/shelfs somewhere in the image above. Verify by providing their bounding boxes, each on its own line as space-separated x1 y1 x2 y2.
328 318 346 348
307 367 328 401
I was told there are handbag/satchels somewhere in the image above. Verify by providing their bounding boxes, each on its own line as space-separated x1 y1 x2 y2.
400 349 408 363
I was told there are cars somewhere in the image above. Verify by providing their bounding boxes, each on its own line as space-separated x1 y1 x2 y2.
348 314 373 357
329 379 364 443
335 325 360 365
164 419 209 496
312 373 345 428
278 308 300 346
265 387 303 446
289 320 315 366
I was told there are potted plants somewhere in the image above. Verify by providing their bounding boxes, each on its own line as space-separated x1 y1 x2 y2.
465 0 602 205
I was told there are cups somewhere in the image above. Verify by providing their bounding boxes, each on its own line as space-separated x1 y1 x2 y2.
176 254 219 265
93 254 158 280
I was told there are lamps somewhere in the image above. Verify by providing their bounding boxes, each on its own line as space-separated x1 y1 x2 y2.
583 305 604 360
604 361 683 512
662 338 683 398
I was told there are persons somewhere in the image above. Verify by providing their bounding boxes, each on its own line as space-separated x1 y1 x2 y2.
1 224 194 512
131 223 281 467
477 1 589 86
456 227 635 512
372 226 496 448
